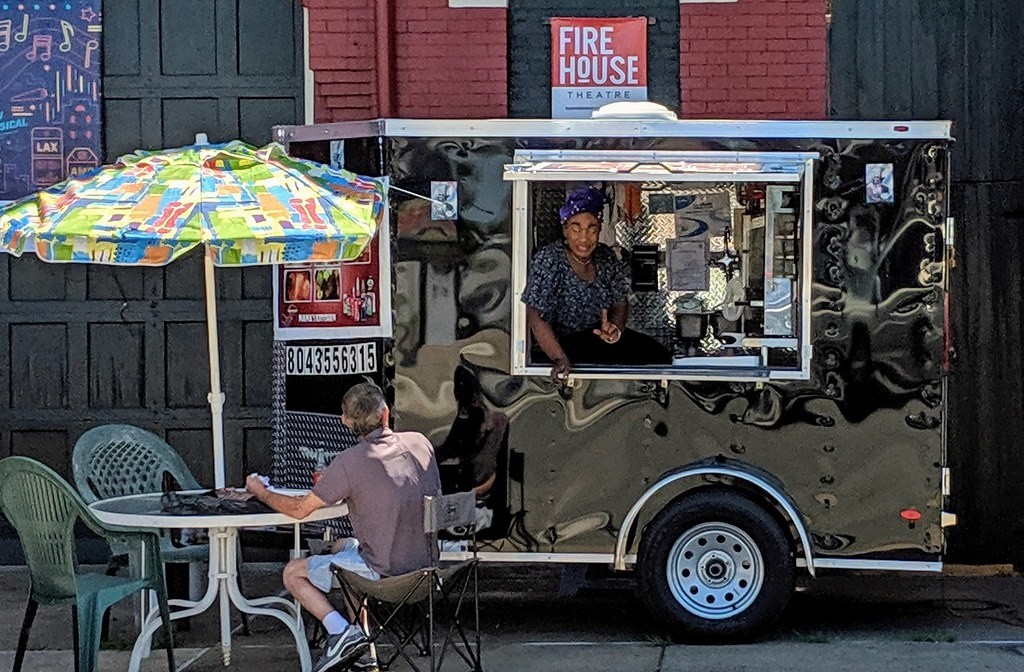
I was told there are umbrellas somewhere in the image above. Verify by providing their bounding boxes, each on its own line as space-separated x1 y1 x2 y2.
0 131 389 494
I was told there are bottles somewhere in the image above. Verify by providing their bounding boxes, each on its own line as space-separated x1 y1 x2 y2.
313 448 327 485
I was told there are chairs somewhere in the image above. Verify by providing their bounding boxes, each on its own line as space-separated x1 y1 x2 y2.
0 426 245 672
306 489 483 672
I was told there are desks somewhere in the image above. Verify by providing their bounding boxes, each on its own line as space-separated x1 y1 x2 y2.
87 488 351 672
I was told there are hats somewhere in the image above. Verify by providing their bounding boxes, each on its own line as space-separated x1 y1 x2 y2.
559 187 605 225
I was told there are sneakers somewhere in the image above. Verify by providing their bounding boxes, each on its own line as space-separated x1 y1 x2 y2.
311 624 379 672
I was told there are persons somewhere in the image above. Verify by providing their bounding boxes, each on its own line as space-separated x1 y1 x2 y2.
521 187 630 385
247 384 442 672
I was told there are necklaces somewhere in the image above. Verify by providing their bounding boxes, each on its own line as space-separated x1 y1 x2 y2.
572 252 591 265
